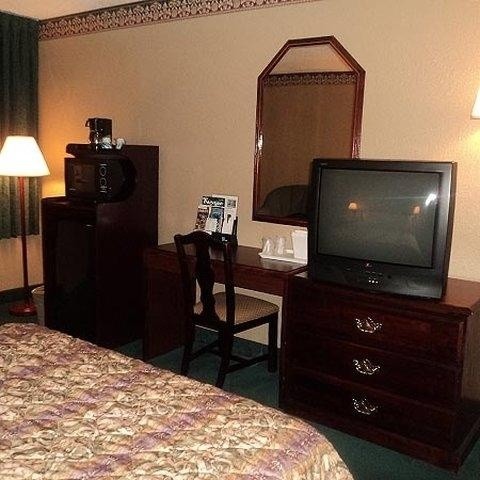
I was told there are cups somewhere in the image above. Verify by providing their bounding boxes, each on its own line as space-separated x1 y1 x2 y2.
291 229 307 259
263 236 286 256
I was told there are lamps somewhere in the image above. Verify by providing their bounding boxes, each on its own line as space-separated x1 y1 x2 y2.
0 134 51 316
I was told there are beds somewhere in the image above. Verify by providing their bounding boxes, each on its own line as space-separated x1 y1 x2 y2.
0 320 356 480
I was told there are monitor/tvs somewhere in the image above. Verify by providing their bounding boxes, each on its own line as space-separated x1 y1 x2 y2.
307 157 458 301
64 157 136 204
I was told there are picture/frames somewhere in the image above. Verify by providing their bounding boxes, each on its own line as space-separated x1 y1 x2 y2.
250 35 365 228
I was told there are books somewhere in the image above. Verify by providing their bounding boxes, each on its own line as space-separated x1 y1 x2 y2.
193 193 239 246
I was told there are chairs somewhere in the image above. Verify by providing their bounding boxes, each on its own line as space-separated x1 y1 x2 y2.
173 231 281 388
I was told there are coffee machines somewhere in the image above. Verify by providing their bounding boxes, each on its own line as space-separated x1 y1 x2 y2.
86 118 111 152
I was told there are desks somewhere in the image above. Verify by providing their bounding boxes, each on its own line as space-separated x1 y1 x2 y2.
143 240 308 408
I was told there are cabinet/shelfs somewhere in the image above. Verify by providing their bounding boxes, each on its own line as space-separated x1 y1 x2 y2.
39 143 160 349
282 270 480 472
